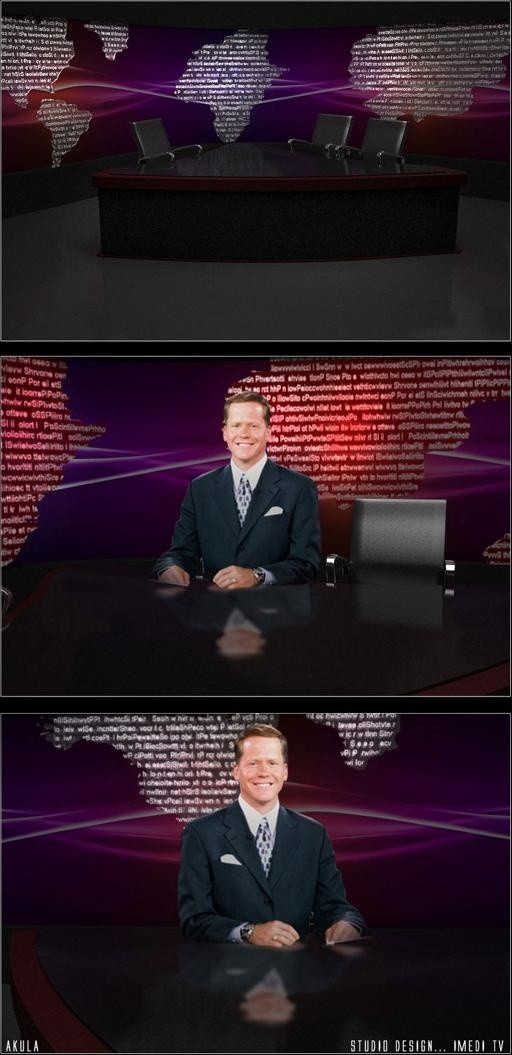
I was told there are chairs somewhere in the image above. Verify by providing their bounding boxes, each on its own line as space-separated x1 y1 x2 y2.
130 117 203 165
334 117 408 165
324 497 456 585
288 113 353 159
194 555 207 579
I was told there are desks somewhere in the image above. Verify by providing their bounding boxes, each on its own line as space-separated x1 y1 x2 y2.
90 137 468 264
2 554 510 699
7 926 508 1051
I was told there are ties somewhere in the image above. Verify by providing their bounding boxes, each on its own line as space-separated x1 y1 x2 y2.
236 475 251 528
256 819 272 878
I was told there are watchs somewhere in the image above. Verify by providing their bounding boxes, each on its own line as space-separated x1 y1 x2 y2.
240 922 256 945
252 568 265 586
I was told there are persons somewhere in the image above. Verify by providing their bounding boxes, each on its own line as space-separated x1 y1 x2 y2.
178 724 365 948
188 941 367 1025
155 392 321 589
152 582 321 668
